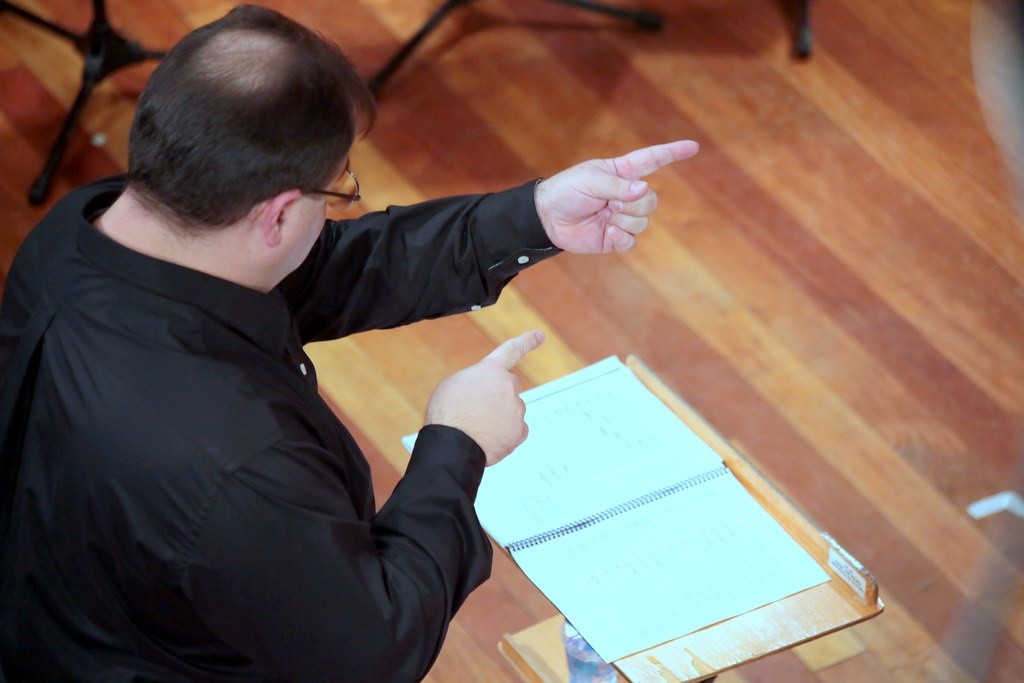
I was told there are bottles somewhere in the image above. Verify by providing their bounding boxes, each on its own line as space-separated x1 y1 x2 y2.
561 619 619 683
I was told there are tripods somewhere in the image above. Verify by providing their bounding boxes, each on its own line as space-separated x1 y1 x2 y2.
0 0 180 207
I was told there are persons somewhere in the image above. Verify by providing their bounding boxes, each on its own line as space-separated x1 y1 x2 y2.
0 5 698 683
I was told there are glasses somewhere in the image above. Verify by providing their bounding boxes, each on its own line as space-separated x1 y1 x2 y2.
311 169 361 212
